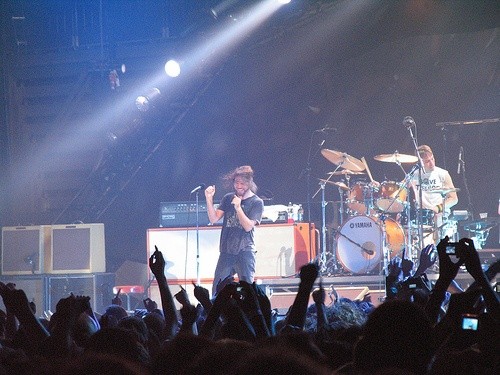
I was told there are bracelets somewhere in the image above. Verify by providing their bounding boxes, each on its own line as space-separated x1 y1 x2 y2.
437 203 443 213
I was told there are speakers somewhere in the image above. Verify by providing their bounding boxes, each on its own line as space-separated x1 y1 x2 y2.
0 275 50 320
45 271 116 318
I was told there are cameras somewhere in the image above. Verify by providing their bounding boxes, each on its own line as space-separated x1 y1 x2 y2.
235 286 243 293
406 283 418 289
445 242 462 255
461 314 480 332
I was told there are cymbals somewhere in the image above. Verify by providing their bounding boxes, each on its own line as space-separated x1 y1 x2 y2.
317 178 350 190
426 187 462 194
320 148 366 172
469 215 500 227
374 154 420 164
323 169 367 175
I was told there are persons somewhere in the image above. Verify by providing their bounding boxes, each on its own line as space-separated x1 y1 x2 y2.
0 235 500 375
410 145 458 248
205 166 264 304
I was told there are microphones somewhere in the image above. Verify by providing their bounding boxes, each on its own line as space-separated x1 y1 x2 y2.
191 183 205 194
410 163 420 176
312 127 337 133
457 150 461 175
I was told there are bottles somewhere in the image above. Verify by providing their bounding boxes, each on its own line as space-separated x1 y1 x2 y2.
287 202 293 219
298 206 304 222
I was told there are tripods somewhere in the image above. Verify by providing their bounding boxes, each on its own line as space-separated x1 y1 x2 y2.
281 134 441 277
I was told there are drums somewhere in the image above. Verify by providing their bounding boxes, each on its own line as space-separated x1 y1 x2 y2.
334 213 405 275
346 179 375 214
375 180 409 214
